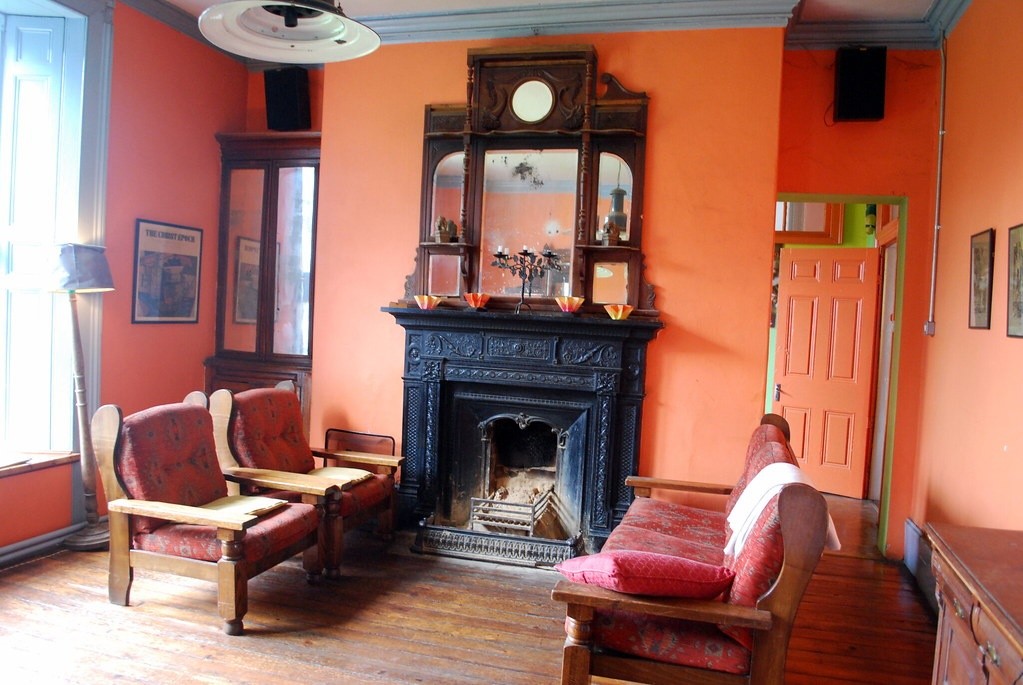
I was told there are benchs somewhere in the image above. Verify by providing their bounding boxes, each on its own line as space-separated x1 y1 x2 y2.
551 413 829 685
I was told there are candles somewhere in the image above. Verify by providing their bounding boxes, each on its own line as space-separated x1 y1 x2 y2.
523 245 528 251
504 248 510 255
530 248 534 253
497 246 503 253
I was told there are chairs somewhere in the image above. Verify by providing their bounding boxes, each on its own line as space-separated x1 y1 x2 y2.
90 390 336 636
208 379 405 580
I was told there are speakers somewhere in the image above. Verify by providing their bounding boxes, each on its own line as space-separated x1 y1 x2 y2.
263 66 312 130
833 45 886 123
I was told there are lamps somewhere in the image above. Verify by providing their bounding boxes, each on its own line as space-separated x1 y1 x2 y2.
58 243 116 552
198 0 380 65
604 156 627 231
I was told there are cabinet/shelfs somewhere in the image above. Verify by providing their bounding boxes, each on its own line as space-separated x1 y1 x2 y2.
923 523 1023 685
202 132 321 447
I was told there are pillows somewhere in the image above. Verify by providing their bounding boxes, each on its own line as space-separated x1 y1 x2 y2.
554 550 735 597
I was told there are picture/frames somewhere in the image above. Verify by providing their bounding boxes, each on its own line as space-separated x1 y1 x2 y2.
968 228 993 331
1006 224 1023 339
233 236 280 326
130 218 204 325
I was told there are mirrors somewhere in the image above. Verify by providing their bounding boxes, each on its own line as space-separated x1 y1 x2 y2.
401 43 658 320
